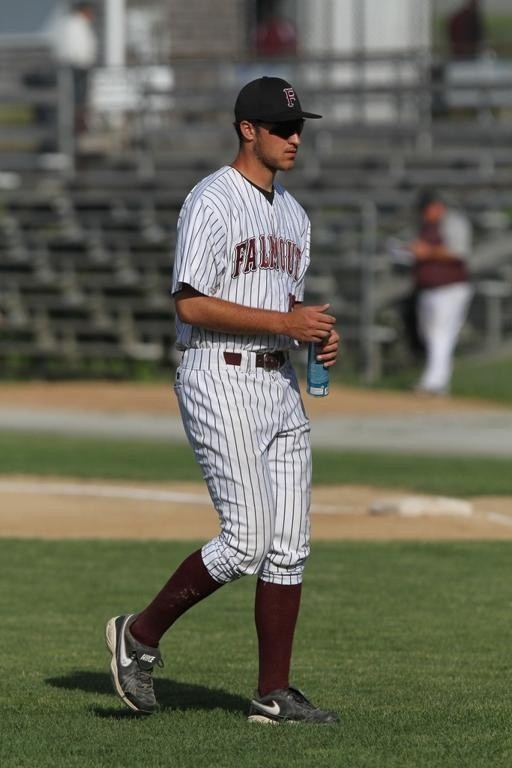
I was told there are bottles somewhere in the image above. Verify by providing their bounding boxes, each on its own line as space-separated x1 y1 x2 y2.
306 340 330 397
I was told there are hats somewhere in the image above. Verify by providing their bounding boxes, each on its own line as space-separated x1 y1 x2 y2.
235 78 323 124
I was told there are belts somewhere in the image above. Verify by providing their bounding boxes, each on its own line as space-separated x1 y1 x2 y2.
222 349 288 372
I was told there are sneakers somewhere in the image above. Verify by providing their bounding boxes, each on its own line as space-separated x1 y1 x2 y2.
104 615 162 716
248 686 336 722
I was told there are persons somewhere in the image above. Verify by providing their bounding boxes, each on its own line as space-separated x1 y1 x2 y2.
107 75 340 725
405 189 477 396
